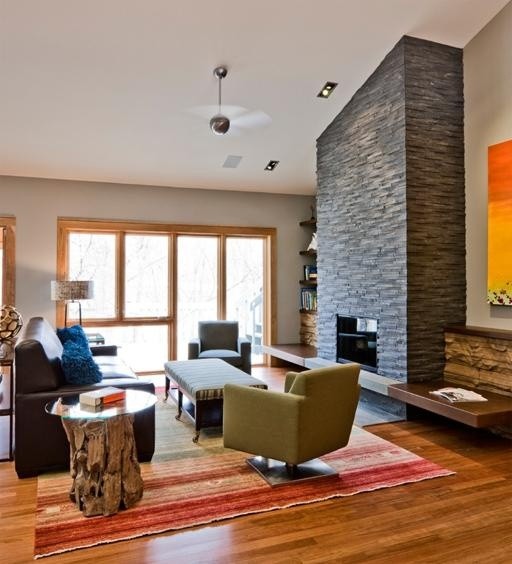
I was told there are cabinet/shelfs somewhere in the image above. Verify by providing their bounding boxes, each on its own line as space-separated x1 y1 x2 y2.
299 205 320 316
0 358 17 465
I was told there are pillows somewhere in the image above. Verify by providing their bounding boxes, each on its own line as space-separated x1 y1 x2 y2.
53 324 93 358
61 339 104 385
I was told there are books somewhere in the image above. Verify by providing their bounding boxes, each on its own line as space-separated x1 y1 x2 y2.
429 385 489 403
80 399 126 412
79 384 127 407
298 222 318 311
86 335 105 342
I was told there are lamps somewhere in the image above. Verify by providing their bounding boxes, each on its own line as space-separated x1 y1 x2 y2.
48 278 95 336
210 66 230 135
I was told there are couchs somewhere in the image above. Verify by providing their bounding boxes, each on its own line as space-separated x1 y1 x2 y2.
187 319 252 376
221 361 368 487
13 314 158 479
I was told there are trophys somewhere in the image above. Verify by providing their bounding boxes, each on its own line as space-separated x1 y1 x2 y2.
310 204 315 220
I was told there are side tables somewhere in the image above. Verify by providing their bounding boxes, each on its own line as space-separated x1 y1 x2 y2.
37 388 159 517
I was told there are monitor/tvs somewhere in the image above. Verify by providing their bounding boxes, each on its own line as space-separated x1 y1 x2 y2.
336 315 379 372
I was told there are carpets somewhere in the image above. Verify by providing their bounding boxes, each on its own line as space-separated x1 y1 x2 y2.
32 372 460 560
